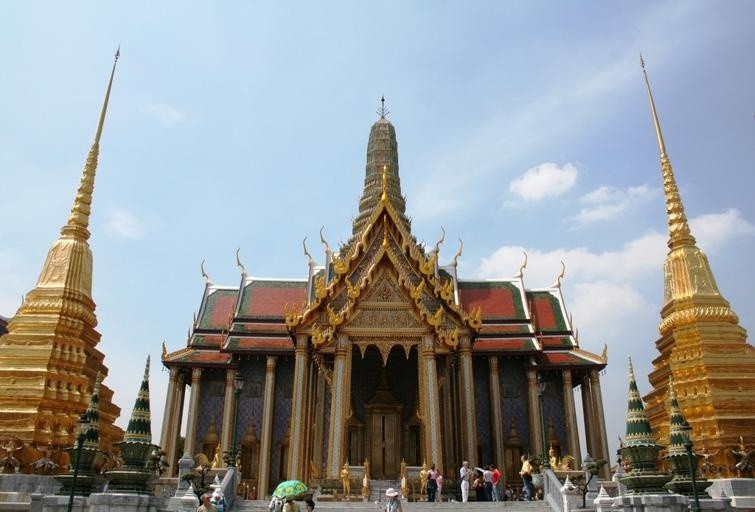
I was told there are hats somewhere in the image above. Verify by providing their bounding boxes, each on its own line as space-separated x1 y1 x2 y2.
385 488 398 497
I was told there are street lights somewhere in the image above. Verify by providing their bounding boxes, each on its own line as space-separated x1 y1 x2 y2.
532 373 550 469
66 413 93 512
676 413 702 512
225 370 245 468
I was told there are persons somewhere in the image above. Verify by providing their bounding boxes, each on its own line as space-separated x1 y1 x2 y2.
29 441 64 475
268 495 287 511
459 460 473 502
282 494 301 512
483 466 492 501
434 468 444 502
697 445 726 479
519 453 534 500
306 499 315 512
212 446 224 468
0 440 25 474
488 463 502 502
504 483 515 500
548 449 557 468
472 470 484 501
425 463 439 501
419 464 429 500
728 441 755 479
383 487 403 512
339 464 351 499
196 493 219 512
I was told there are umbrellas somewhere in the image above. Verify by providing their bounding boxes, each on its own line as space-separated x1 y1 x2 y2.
272 479 309 498
474 466 485 472
